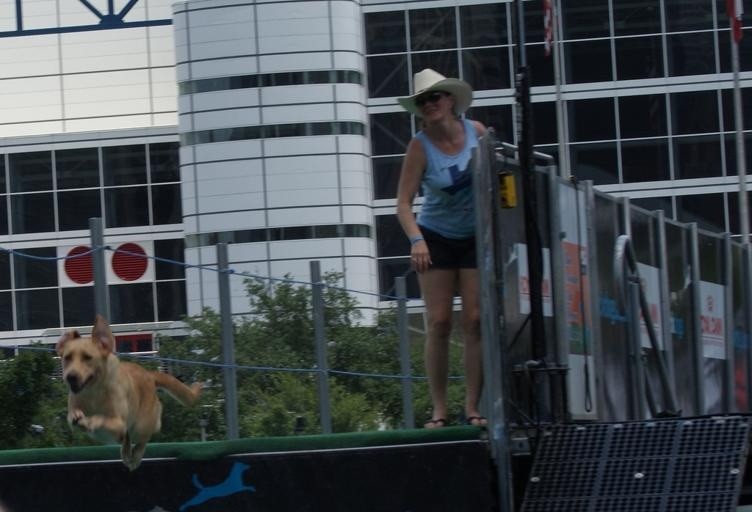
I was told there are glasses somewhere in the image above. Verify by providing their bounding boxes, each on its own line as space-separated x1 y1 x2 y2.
415 93 440 106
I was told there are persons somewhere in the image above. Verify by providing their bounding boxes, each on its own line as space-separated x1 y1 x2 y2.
397 63 496 430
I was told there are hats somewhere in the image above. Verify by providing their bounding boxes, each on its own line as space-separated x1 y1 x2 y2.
397 69 472 119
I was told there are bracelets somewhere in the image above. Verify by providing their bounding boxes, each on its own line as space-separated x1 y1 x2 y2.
409 233 425 246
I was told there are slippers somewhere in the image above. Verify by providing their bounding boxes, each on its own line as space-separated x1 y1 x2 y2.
424 419 447 429
465 416 487 425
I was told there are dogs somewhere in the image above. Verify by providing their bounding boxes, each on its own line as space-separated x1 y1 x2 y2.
55 314 202 471
